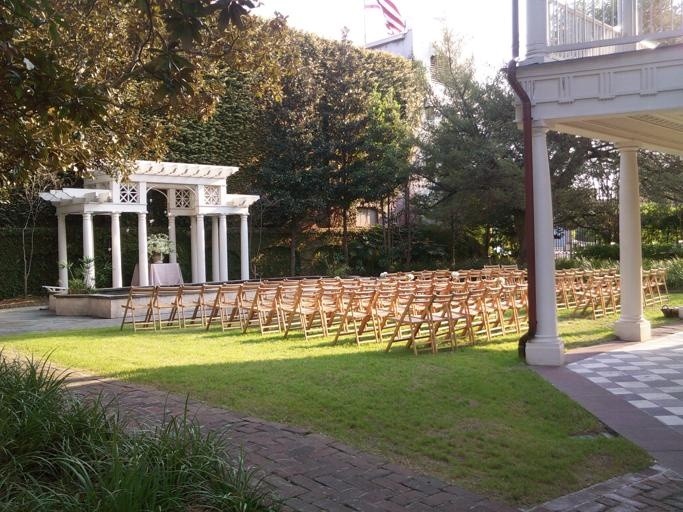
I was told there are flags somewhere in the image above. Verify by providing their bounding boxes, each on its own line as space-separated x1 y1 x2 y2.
374 0 406 36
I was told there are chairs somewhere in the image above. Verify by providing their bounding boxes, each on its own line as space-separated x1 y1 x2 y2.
120 265 670 356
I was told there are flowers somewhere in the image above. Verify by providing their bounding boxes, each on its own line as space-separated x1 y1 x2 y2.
147 232 175 257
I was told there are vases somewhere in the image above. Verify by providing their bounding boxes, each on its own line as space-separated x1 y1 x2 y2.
152 251 165 263
